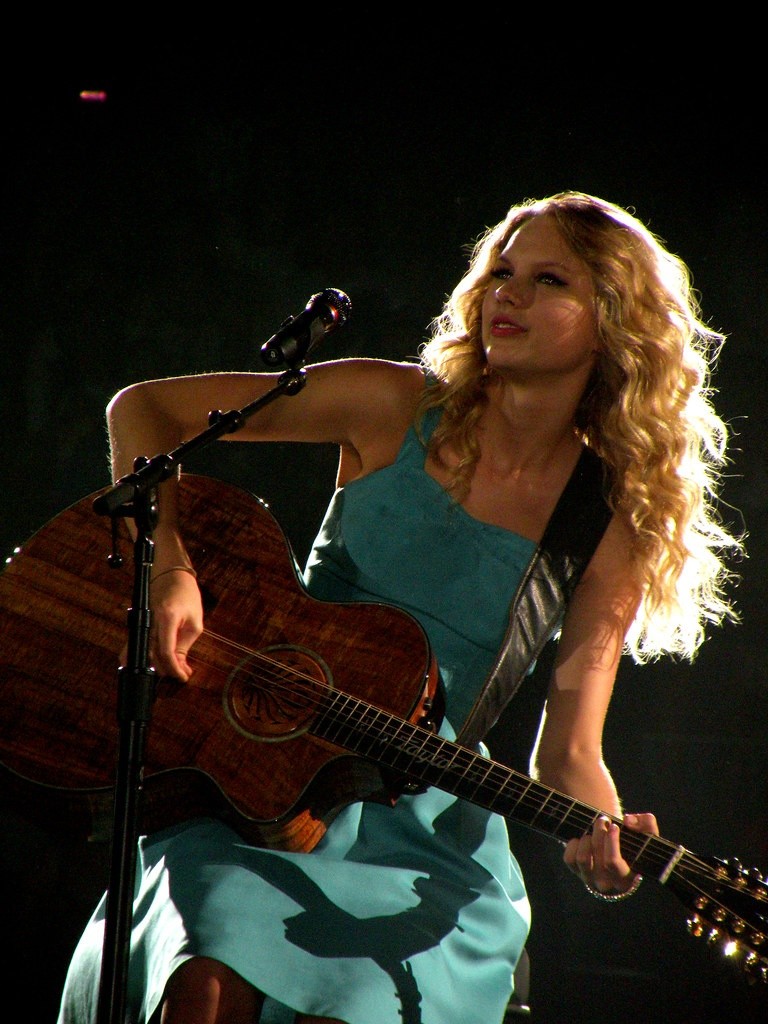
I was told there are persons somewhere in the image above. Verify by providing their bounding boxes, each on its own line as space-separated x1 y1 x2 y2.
58 189 748 1024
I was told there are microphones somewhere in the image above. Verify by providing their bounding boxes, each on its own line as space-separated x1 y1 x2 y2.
258 287 354 371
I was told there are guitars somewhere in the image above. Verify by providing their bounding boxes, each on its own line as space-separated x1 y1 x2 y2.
0 467 767 995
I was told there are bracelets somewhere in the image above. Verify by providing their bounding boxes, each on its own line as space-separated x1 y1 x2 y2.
584 874 643 902
149 566 197 583
